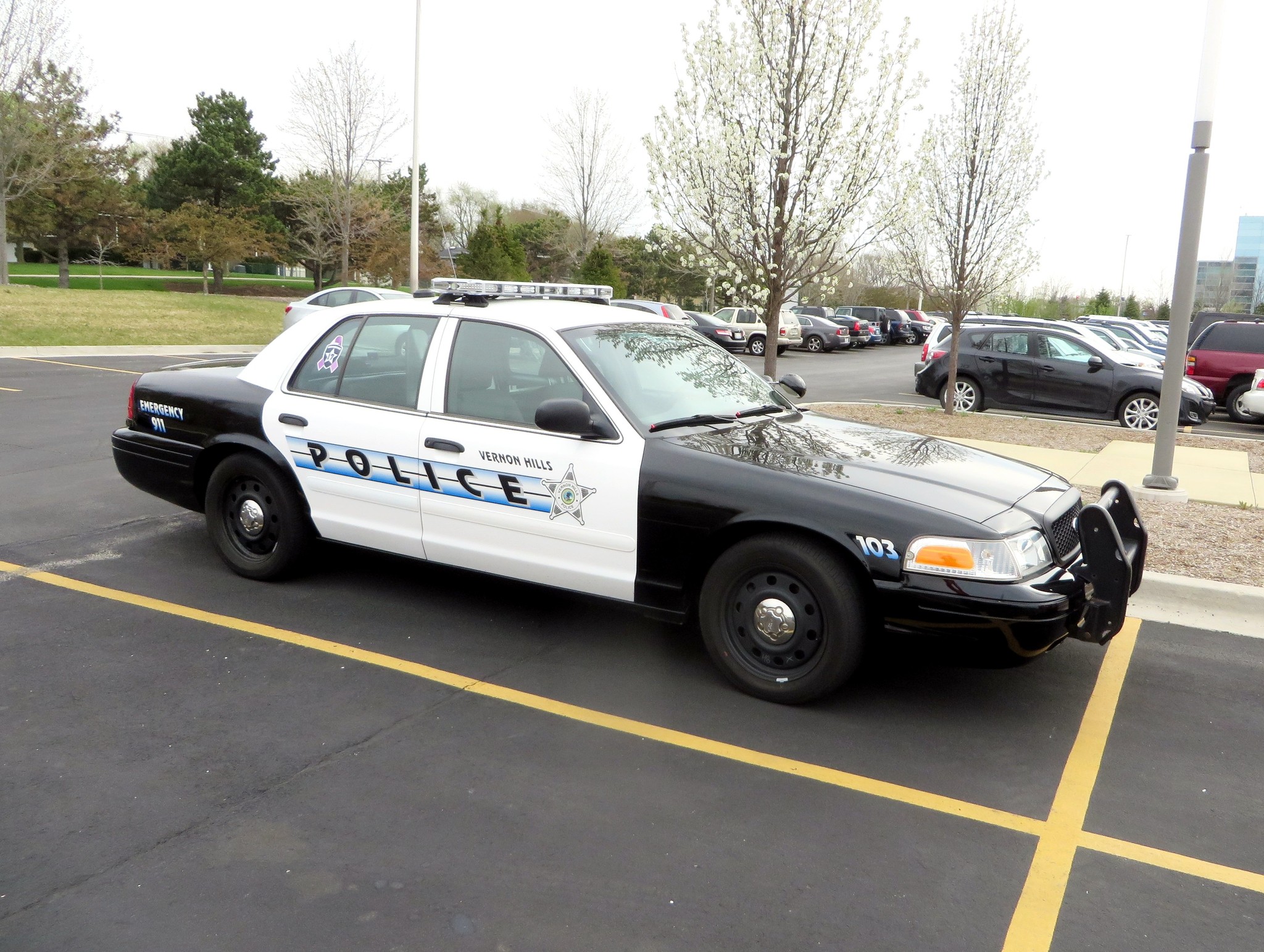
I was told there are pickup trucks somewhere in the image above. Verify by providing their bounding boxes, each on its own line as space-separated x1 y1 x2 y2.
789 305 871 350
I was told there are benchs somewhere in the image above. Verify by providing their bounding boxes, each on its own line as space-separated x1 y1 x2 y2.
324 372 407 408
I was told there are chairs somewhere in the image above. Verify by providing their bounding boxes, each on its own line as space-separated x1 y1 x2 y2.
524 348 584 425
456 350 524 424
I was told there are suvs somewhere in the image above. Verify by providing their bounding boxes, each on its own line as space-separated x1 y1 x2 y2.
610 298 691 329
711 306 803 356
1184 319 1264 424
834 306 891 340
878 307 1169 377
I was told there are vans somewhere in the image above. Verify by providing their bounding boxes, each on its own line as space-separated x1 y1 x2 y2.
1186 311 1264 353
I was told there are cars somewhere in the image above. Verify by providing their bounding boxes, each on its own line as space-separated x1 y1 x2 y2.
282 287 414 357
683 310 747 354
788 314 850 353
111 277 1151 707
914 326 1217 431
836 315 882 347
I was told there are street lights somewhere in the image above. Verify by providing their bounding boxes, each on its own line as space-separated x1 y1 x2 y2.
1117 234 1132 316
